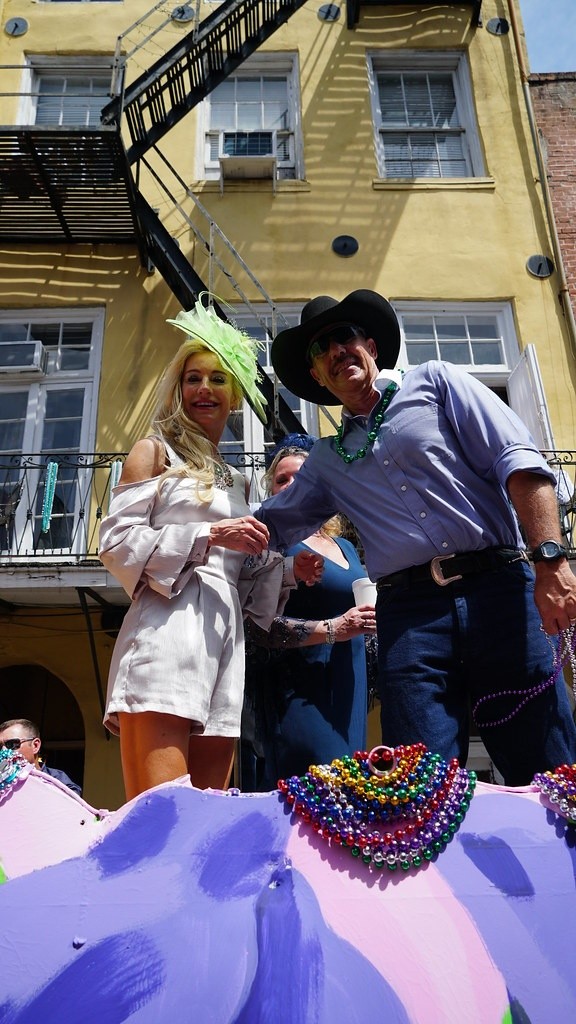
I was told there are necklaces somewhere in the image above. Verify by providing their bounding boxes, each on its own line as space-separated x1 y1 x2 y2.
531 763 576 823
278 743 476 870
472 620 576 729
334 369 404 463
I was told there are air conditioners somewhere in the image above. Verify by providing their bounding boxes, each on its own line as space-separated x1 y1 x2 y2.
0 340 49 380
218 128 279 199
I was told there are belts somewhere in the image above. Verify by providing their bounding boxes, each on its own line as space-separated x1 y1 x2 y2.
376 544 530 594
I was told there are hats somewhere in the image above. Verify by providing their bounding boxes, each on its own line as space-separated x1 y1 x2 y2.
164 290 269 425
271 289 401 405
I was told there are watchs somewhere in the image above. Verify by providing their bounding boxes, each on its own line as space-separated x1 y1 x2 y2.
532 540 568 564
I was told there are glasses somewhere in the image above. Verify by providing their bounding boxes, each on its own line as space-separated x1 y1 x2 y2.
308 324 366 359
0 737 36 751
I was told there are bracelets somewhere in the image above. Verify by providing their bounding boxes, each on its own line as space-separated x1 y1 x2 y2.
324 619 335 645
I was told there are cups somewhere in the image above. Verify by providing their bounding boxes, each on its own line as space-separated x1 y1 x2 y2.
351 576 378 609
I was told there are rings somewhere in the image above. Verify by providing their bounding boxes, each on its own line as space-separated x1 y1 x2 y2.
365 619 367 625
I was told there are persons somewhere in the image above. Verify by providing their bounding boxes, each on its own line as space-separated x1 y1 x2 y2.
255 288 575 783
0 720 81 796
244 433 376 774
98 309 271 802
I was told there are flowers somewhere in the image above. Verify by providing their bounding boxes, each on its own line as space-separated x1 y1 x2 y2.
208 312 267 380
